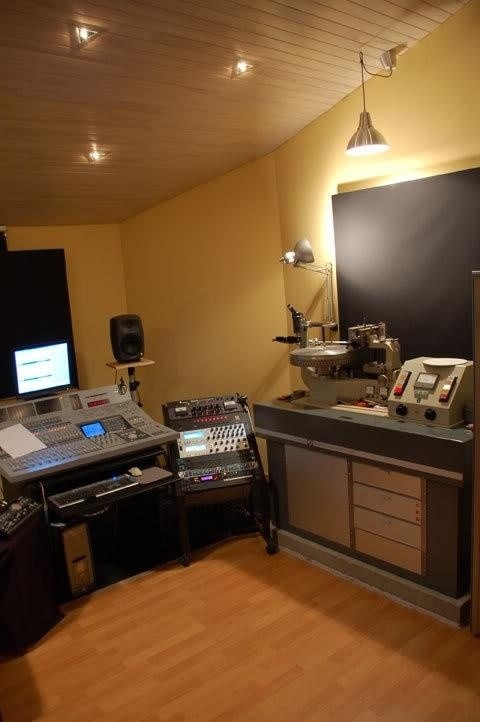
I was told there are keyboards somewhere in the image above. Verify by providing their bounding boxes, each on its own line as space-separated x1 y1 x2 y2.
47 472 139 509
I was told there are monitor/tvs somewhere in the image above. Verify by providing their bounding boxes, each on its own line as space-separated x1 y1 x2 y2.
11 339 74 397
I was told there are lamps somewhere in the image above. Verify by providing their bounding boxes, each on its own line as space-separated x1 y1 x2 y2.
280 239 335 325
343 49 388 158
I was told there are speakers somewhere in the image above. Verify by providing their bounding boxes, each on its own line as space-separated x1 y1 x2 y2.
110 314 144 362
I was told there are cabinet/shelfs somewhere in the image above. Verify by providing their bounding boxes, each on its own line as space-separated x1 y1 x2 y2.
265 438 470 601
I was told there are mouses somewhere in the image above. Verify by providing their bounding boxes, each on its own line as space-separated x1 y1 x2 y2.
127 466 143 477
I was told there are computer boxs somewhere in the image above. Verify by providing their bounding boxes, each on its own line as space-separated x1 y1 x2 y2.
42 516 97 601
